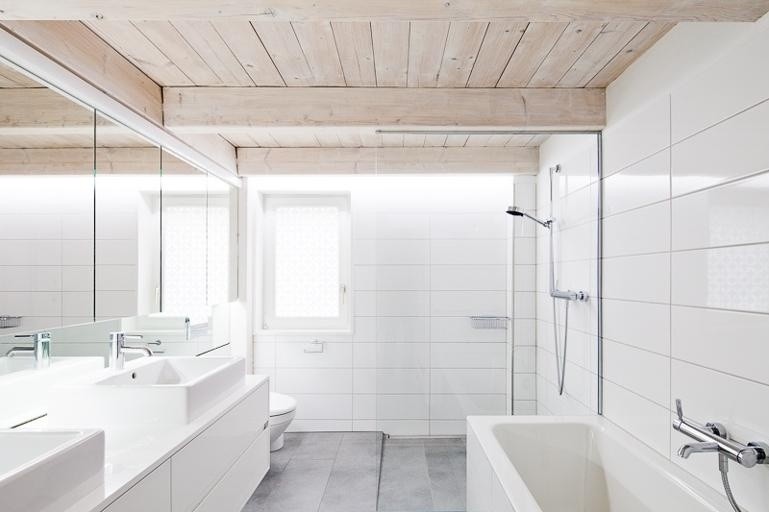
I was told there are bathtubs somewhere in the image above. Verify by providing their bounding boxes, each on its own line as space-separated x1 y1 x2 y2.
464 415 747 512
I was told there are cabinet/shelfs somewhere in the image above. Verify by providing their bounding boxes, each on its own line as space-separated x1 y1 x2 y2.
172 376 271 512
93 459 171 512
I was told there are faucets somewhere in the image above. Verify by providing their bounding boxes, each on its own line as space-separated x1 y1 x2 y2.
670 398 760 474
108 331 152 372
5 332 51 369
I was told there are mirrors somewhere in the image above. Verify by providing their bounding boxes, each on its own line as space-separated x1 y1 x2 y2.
161 143 208 324
95 106 161 357
208 169 239 313
0 55 96 429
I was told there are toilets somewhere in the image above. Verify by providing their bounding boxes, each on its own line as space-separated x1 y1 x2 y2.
269 393 296 453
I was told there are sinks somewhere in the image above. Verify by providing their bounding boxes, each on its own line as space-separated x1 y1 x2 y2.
82 355 246 426
0 354 104 386
0 426 104 512
